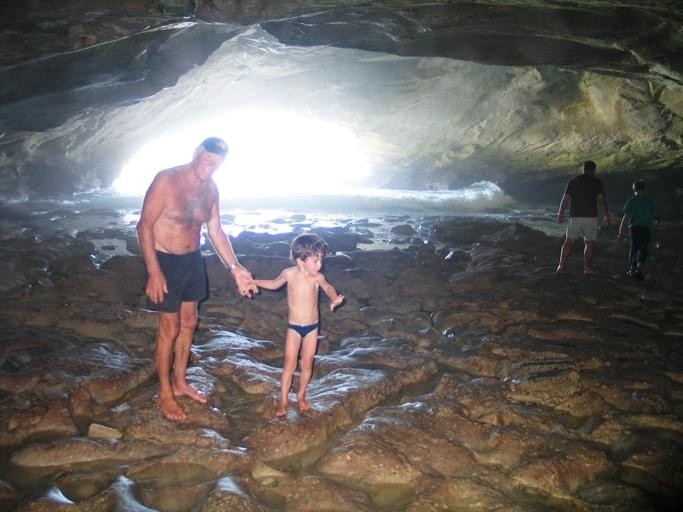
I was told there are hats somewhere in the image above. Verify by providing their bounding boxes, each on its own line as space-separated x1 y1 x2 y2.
201 136 229 156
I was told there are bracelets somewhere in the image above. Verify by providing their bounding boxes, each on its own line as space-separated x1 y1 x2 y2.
223 259 240 271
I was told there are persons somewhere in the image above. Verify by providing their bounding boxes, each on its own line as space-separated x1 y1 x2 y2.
246 234 344 417
617 179 660 282
552 160 611 274
137 138 261 421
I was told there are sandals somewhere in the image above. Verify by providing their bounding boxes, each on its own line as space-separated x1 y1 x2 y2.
626 268 643 281
